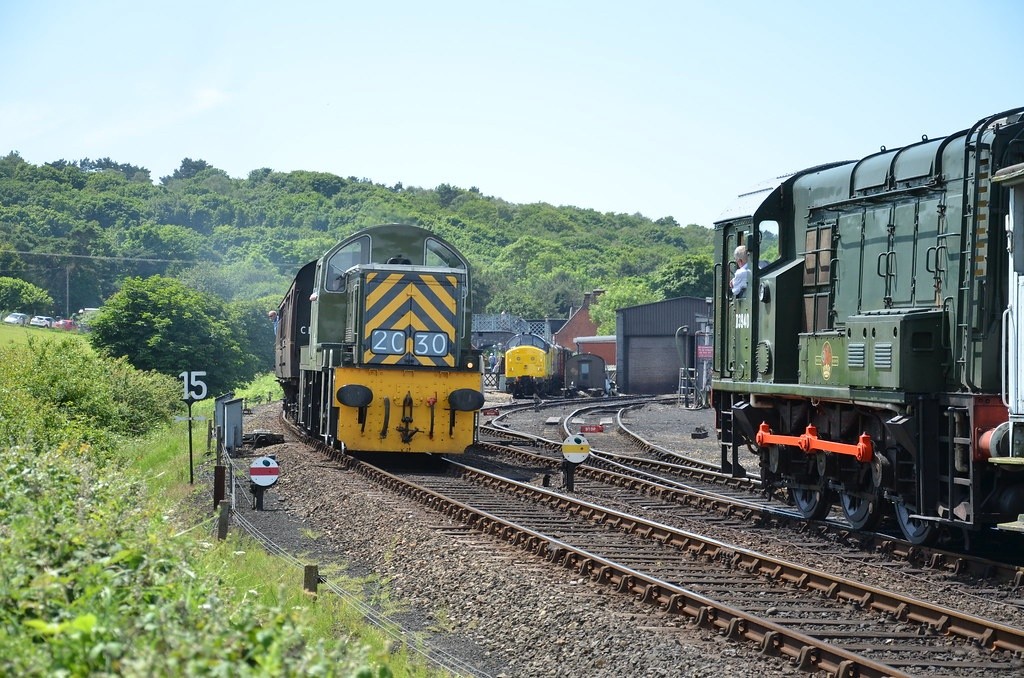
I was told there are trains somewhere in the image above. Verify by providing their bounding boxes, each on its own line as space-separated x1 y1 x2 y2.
711 106 1024 544
503 333 572 397
274 224 485 455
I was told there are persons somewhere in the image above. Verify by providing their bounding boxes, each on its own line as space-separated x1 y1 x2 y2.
309 270 346 302
500 309 528 333
488 352 498 373
269 310 281 335
729 245 770 297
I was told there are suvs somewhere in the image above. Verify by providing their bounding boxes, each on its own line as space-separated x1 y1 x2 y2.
30 315 57 329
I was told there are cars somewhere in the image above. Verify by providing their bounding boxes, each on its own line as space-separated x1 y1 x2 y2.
5 313 30 325
53 319 78 331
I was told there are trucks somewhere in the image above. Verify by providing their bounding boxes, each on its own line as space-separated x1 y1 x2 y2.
75 308 99 320
